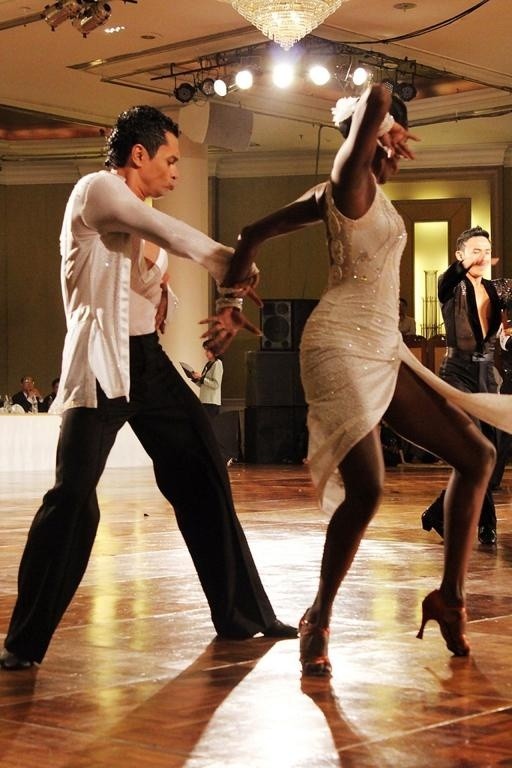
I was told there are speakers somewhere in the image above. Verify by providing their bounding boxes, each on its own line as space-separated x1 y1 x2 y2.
260 297 291 351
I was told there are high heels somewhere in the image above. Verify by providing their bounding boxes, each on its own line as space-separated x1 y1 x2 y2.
416 590 472 655
298 608 333 676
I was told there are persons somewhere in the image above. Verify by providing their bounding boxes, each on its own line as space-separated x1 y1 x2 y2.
43 379 60 412
399 297 415 336
190 339 234 468
12 375 45 413
197 83 512 674
0 105 301 671
422 225 512 545
489 328 512 490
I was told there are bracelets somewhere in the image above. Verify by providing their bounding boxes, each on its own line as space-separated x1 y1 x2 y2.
213 297 244 312
376 114 395 137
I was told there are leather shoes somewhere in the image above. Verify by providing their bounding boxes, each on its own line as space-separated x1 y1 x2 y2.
421 506 445 536
0 646 35 670
261 620 294 637
475 525 496 547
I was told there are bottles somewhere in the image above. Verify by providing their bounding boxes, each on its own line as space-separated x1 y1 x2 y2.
30 397 39 415
3 396 12 416
